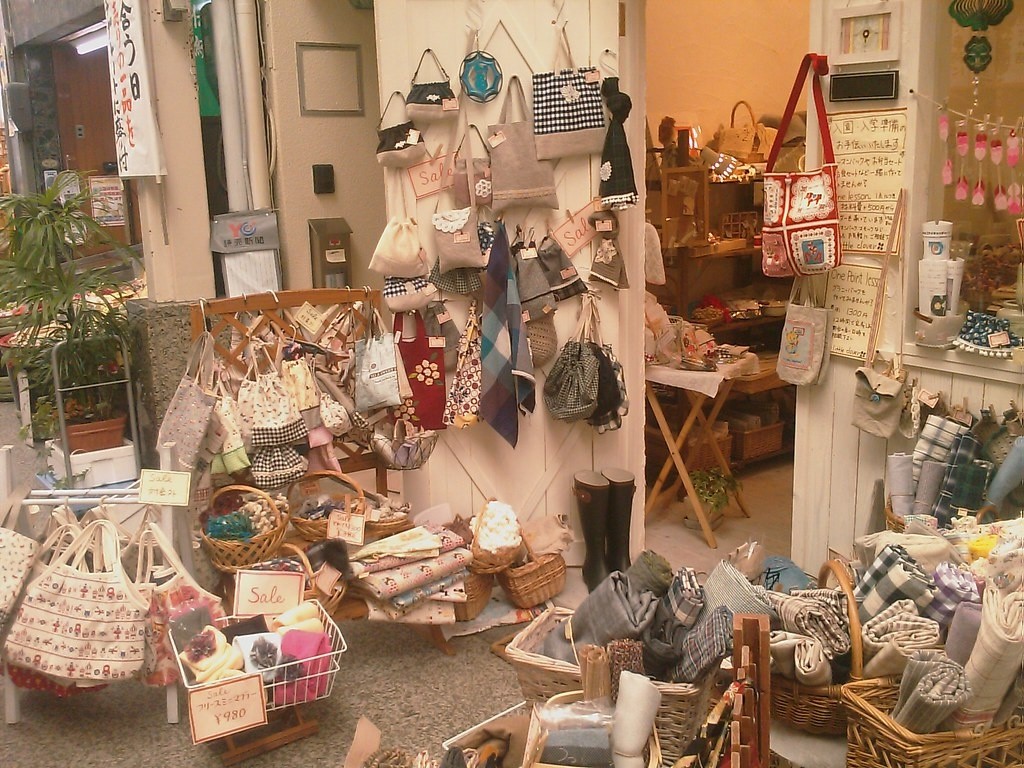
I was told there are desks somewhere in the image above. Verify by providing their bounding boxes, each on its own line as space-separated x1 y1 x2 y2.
644 363 753 547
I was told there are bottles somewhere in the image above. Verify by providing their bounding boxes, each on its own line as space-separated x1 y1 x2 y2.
996 300 1024 338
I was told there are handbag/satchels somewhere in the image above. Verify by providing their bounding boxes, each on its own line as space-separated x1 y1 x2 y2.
156 18 632 488
775 273 833 386
0 483 229 697
717 100 777 162
762 51 841 277
851 365 904 440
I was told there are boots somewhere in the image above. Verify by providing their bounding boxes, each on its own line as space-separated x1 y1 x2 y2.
574 467 637 596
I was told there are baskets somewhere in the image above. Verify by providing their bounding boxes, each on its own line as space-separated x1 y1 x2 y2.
647 313 793 474
443 507 1024 768
199 467 566 622
170 597 347 712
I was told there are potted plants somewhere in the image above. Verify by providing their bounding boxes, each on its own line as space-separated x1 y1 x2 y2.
1 171 140 454
684 467 743 528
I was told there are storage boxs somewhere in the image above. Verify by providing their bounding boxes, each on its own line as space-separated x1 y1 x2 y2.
45 438 138 489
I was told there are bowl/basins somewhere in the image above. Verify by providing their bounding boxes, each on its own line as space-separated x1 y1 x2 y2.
761 304 784 316
